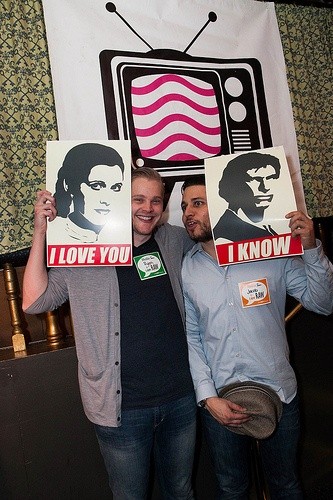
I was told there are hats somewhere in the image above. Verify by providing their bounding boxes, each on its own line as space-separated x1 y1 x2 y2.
217 382 284 441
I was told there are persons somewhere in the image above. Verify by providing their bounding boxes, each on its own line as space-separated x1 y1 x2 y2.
21 167 200 500
180 174 333 500
